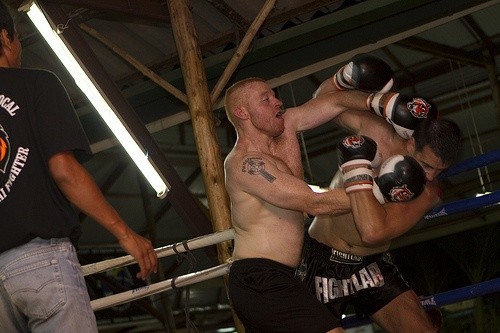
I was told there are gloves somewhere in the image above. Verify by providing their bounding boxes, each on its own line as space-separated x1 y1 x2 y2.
336 135 378 192
333 54 394 95
373 155 427 205
368 90 436 139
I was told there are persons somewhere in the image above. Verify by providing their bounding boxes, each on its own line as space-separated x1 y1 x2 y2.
0 1 160 333
294 54 463 333
222 76 438 333
